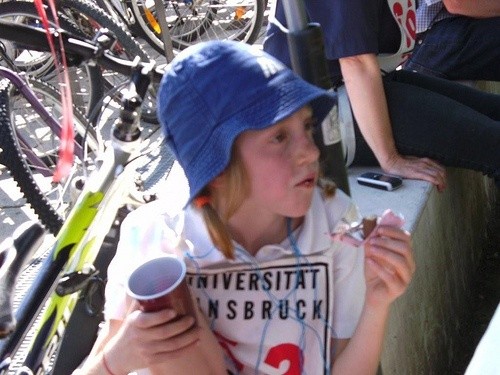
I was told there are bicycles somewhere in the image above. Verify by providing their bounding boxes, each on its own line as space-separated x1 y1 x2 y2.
0 0 351 375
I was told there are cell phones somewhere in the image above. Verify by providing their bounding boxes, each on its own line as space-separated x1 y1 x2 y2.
357 171 402 191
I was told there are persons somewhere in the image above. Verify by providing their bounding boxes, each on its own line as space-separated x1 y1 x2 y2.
68 39 414 375
258 1 500 191
392 0 500 83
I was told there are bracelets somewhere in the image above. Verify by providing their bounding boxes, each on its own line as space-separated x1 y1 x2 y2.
102 348 115 375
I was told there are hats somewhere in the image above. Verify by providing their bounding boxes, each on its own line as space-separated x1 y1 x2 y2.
156 40 337 209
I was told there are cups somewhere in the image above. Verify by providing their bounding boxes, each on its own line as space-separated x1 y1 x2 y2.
126 255 200 337
